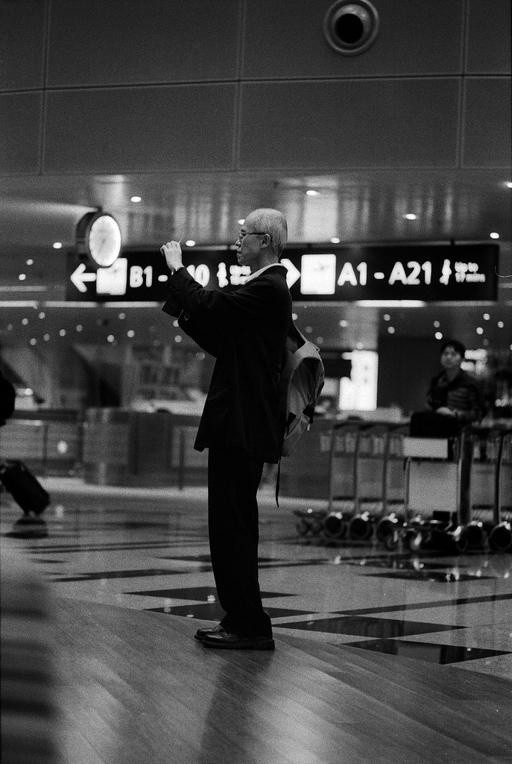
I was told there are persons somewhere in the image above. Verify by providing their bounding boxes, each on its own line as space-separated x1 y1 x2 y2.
159 208 293 650
427 339 483 411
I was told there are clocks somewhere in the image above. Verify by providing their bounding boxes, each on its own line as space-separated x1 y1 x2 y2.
75 207 122 270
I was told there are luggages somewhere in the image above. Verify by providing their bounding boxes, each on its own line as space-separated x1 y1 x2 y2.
0 458 49 517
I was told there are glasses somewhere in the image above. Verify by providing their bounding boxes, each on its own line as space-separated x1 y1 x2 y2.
238 231 266 238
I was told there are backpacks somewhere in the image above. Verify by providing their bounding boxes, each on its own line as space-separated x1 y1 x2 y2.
284 322 324 458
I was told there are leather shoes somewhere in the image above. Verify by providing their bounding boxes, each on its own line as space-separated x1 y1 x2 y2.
194 625 275 652
